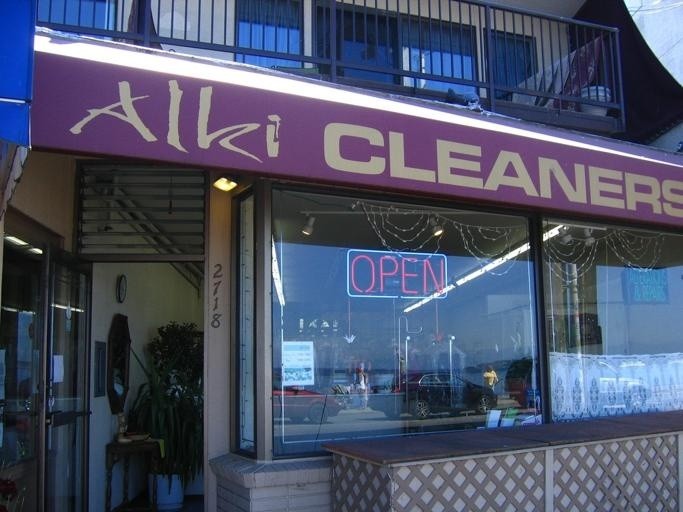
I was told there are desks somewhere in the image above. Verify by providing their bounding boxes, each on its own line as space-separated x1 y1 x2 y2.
273 408 538 456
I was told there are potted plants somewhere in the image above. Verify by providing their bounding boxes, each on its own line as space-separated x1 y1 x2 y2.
129 318 204 510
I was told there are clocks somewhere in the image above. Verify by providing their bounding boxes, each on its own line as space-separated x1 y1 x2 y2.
118 275 126 302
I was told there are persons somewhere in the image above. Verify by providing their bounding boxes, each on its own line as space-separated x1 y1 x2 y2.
483 364 499 392
356 360 368 409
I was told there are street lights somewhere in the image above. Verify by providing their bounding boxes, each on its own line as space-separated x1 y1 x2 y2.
446 333 456 407
402 335 413 413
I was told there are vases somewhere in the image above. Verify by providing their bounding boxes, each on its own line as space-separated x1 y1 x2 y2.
579 87 611 117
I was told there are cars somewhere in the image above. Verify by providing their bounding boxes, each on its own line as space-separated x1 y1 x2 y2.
367 369 498 419
270 371 344 423
